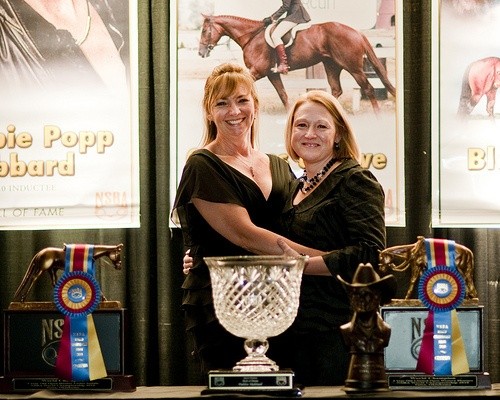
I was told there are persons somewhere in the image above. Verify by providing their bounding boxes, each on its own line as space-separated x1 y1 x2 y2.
182 90 386 386
262 0 311 75
337 263 396 355
171 62 331 385
1 0 135 97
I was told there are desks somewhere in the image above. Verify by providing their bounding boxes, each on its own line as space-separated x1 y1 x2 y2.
0 386 500 400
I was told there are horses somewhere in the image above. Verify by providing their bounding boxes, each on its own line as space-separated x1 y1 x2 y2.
377 237 478 300
198 10 396 113
13 244 124 303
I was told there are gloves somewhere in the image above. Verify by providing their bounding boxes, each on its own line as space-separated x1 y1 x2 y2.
263 17 272 27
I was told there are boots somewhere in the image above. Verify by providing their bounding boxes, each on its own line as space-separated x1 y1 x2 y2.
274 44 289 74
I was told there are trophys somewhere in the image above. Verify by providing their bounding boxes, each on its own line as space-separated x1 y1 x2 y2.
203 254 310 389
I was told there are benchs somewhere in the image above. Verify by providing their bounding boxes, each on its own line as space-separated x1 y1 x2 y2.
285 78 385 113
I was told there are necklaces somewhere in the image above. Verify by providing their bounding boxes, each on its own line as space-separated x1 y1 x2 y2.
75 0 91 48
299 156 338 194
229 152 254 177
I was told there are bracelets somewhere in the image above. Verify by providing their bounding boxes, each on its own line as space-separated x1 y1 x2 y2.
301 253 309 263
